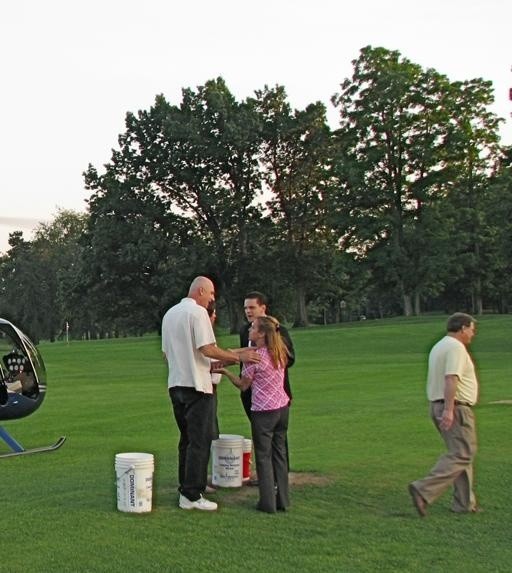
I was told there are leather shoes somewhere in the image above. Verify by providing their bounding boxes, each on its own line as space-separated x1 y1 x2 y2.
247 480 259 486
410 485 426 517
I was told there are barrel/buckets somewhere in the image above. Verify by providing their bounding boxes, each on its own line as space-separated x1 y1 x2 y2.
243 438 254 481
210 434 244 488
114 452 155 513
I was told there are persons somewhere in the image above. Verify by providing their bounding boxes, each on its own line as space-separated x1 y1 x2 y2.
408 312 478 517
210 316 291 515
162 276 262 511
238 292 296 487
204 308 239 444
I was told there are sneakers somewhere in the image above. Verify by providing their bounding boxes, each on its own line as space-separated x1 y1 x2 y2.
179 494 217 511
204 485 216 494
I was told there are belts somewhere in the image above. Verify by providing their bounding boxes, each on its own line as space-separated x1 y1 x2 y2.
435 398 471 407
